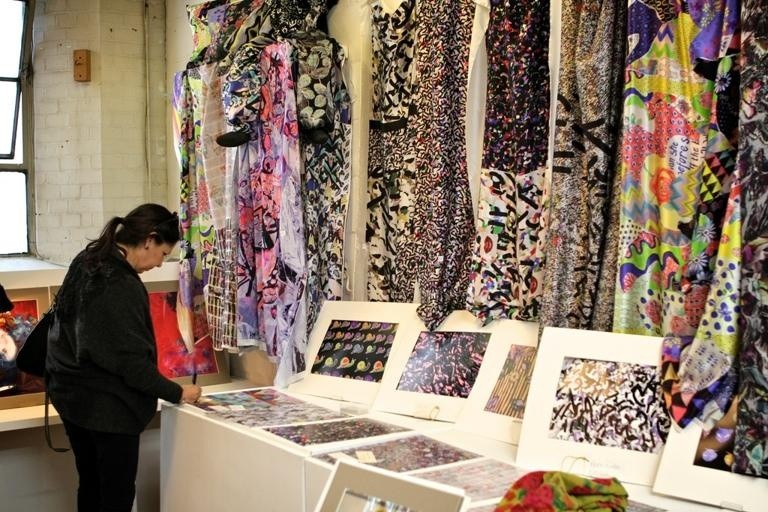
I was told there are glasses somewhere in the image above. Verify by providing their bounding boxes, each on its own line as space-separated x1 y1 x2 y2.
152 212 178 232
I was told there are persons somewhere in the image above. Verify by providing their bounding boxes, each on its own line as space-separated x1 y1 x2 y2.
42 202 202 512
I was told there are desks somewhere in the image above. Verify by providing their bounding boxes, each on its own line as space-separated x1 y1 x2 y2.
158 386 531 512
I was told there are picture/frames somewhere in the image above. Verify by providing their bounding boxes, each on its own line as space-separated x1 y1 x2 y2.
141 282 231 390
0 287 52 412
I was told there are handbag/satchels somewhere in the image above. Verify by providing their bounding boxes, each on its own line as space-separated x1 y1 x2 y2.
16 311 54 377
